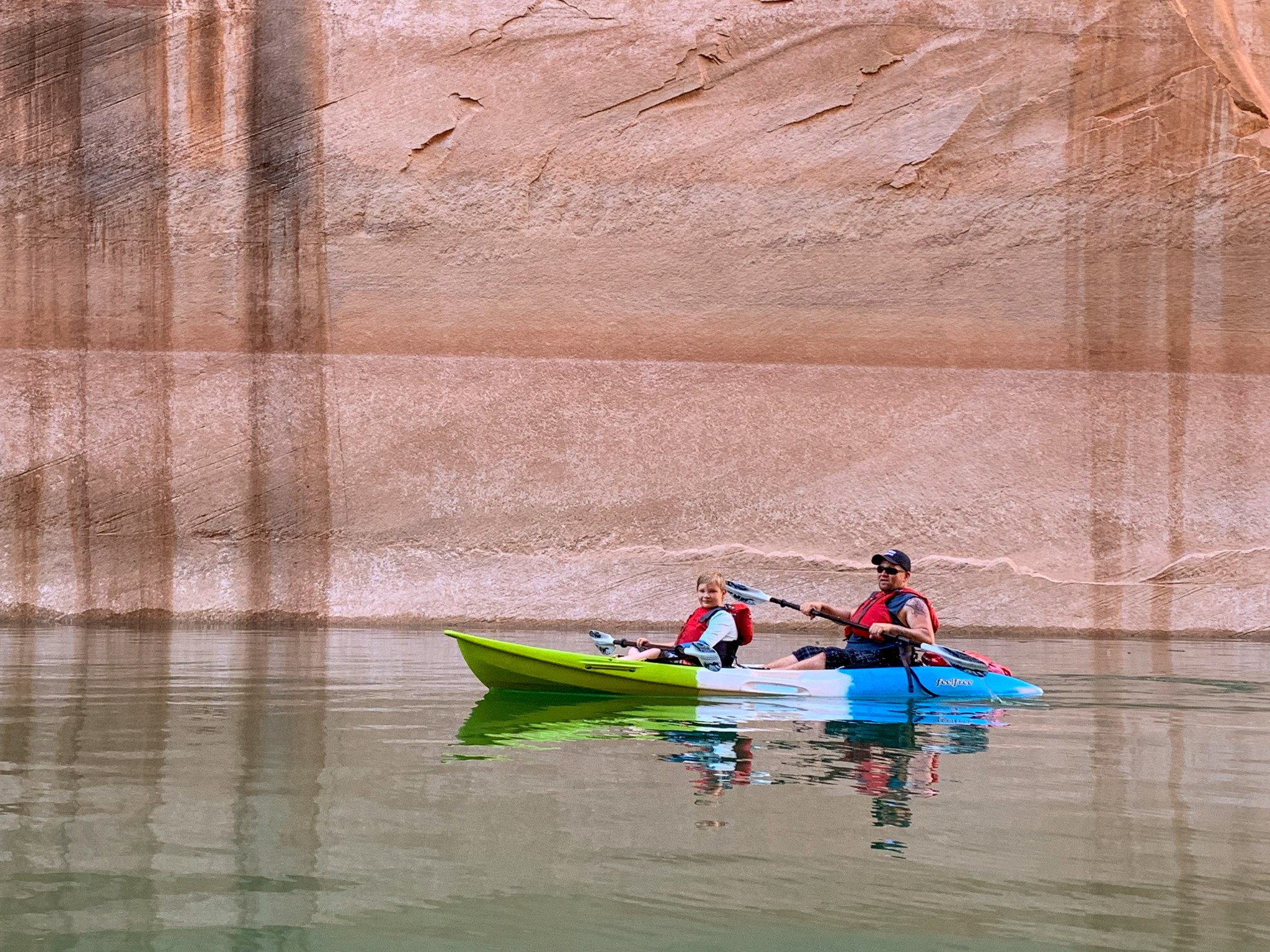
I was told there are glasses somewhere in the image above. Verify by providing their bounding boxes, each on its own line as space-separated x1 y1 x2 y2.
877 566 906 575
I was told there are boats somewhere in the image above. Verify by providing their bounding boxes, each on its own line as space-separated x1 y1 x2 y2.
444 628 1047 707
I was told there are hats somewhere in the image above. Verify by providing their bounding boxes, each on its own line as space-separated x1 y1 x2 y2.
872 549 911 572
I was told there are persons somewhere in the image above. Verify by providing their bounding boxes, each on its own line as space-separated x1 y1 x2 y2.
758 549 939 670
613 569 740 668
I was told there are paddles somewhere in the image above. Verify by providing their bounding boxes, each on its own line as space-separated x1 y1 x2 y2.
588 629 723 673
724 578 989 678
